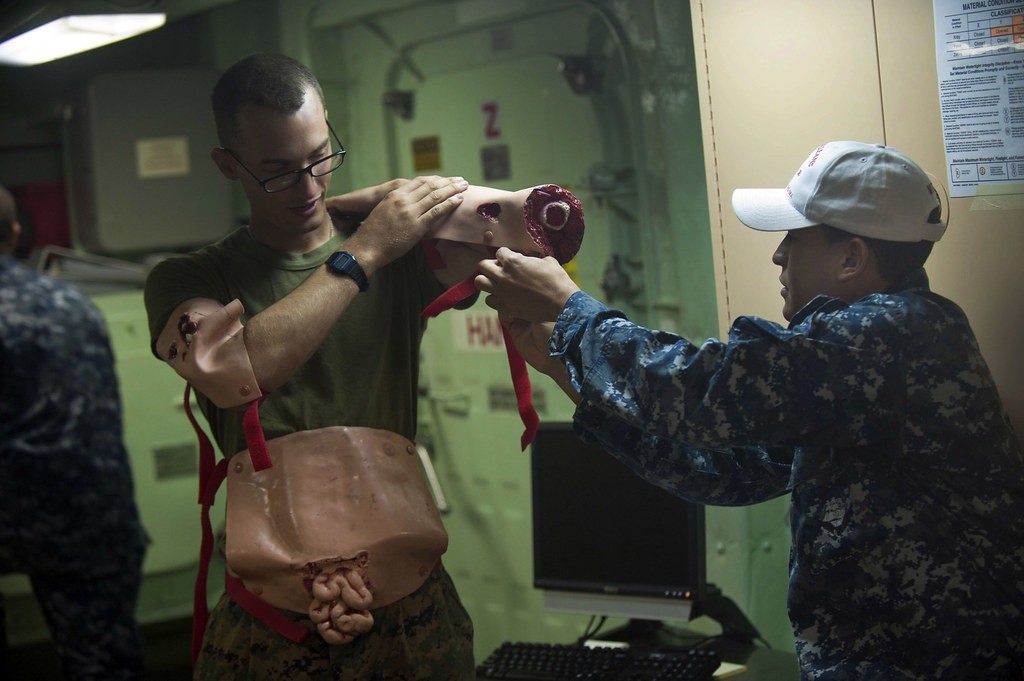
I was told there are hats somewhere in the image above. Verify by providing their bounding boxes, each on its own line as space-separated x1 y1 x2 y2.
730 140 953 243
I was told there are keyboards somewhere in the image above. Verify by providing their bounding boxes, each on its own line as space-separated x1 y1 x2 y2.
477 640 722 681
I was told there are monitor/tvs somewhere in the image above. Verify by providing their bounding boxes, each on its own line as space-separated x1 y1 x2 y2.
531 423 706 623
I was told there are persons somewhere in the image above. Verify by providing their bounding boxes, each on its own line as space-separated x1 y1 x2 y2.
474 141 1023 681
144 53 498 681
0 188 154 681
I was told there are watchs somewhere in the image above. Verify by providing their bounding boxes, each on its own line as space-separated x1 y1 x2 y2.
325 250 370 292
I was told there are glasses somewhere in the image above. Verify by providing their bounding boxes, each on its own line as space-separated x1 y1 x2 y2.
218 118 349 195
